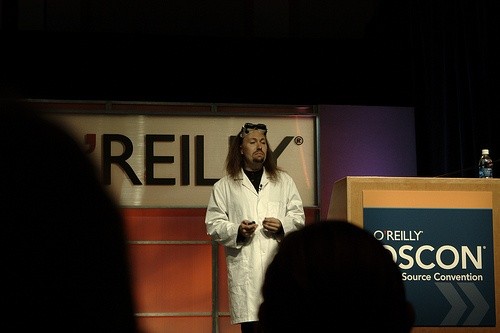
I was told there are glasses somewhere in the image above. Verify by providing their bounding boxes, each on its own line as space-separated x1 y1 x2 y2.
240 122 268 138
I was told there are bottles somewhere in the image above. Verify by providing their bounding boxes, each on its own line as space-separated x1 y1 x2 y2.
479 150 492 178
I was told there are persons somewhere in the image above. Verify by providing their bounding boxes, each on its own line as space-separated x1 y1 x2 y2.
256 219 413 333
205 126 305 333
0 101 140 333
